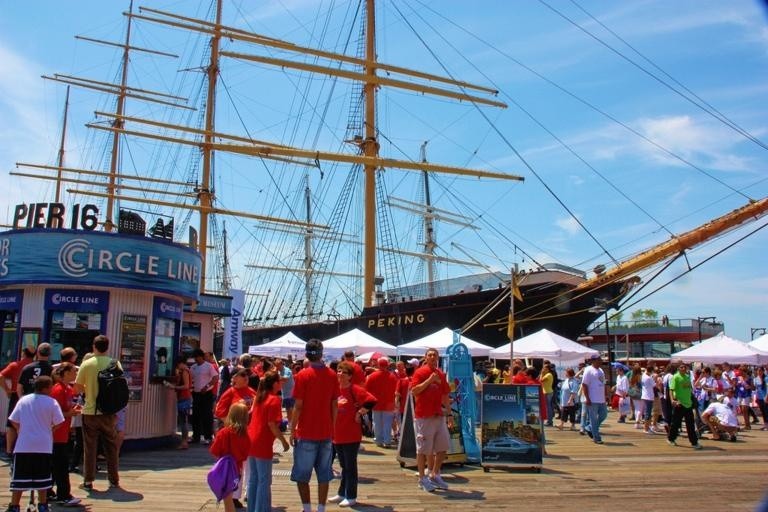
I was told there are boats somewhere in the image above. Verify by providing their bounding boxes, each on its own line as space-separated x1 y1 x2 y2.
483 431 538 459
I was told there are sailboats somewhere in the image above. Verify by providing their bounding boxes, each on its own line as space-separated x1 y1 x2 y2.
0 1 765 382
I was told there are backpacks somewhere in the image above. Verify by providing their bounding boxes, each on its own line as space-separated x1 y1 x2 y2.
95 359 129 417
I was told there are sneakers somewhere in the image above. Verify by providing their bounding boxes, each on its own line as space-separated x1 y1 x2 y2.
430 475 448 488
110 482 120 488
728 436 741 443
176 435 213 451
418 476 435 492
580 426 603 444
545 416 575 431
5 489 81 512
416 468 431 476
665 440 678 446
692 443 700 448
333 470 340 479
374 432 400 448
328 495 344 503
743 418 767 432
1 453 11 461
68 455 106 473
339 499 356 507
78 483 93 490
712 434 723 440
618 416 666 434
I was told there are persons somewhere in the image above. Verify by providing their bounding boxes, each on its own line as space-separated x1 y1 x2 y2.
214 369 288 512
666 362 703 449
291 339 339 512
538 355 768 435
473 359 540 389
329 363 377 507
700 397 738 442
162 347 426 452
1 334 135 512
410 349 451 492
582 355 607 444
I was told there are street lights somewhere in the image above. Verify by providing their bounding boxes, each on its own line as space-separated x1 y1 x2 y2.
697 316 721 369
751 327 767 342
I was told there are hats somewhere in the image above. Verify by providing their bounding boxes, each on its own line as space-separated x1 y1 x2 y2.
592 354 600 358
231 367 250 377
191 349 204 358
377 358 389 366
407 358 420 366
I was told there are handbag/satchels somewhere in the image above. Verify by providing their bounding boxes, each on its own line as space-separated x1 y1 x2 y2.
628 387 641 399
574 403 579 410
361 413 371 435
208 456 240 508
691 393 697 408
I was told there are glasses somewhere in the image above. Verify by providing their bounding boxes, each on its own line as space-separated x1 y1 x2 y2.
239 372 250 377
